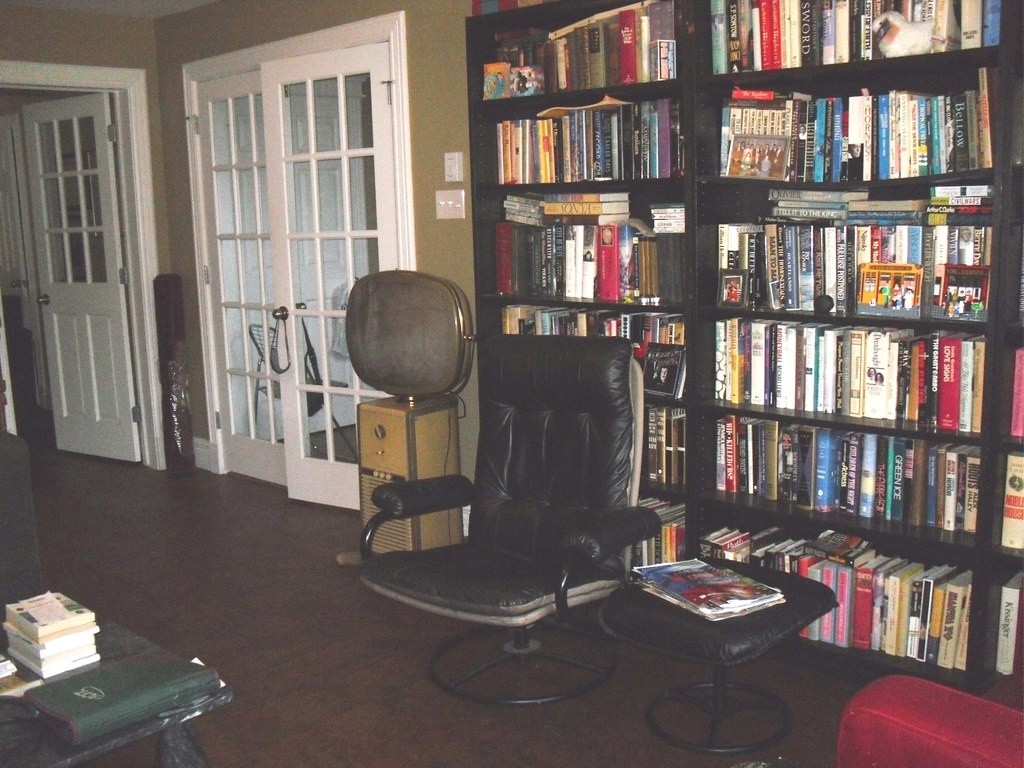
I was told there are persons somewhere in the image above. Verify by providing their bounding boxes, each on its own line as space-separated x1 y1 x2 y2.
798 124 807 140
875 374 883 384
651 366 674 393
867 367 875 380
848 144 863 162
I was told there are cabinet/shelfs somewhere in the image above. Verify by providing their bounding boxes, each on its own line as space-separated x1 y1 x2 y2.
466 0 1024 697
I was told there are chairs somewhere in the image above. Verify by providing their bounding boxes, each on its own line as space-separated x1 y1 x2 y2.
360 334 661 706
249 324 358 463
0 431 235 768
2 294 39 408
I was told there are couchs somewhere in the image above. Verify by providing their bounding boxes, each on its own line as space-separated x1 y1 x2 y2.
837 676 1023 768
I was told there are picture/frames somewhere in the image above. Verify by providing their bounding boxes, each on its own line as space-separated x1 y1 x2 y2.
725 135 792 180
642 342 686 399
718 268 750 307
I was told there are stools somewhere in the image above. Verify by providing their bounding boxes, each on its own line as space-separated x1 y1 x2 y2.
599 556 839 756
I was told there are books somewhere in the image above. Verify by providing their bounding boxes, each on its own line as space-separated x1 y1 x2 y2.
0 590 220 744
480 2 1024 680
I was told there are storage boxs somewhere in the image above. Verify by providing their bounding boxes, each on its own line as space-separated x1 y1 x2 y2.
482 61 545 99
650 40 677 82
